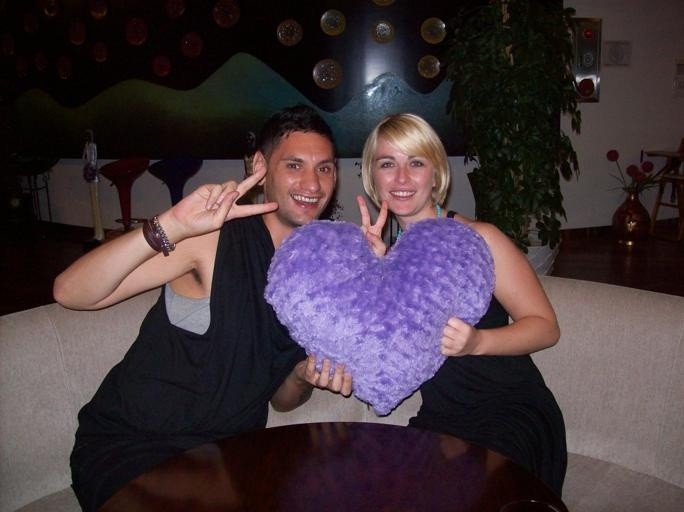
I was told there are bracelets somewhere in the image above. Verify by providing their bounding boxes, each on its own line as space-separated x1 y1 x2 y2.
142 215 174 257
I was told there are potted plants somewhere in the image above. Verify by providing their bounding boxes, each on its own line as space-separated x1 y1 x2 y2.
445 1 586 276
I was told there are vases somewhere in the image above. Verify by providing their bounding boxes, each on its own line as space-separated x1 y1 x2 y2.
606 188 654 249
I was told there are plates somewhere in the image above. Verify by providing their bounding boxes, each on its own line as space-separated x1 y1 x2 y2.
419 18 449 44
368 19 395 44
277 19 302 47
0 2 244 81
417 57 441 79
321 11 346 37
313 59 346 91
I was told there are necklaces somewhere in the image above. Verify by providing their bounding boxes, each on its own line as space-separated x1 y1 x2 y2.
398 204 442 240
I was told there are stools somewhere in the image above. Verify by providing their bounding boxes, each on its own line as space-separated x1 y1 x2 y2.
144 147 206 207
96 153 153 235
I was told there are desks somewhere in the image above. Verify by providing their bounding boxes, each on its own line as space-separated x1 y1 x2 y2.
88 419 570 512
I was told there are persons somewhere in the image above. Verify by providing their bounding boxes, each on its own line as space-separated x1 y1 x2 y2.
50 102 354 511
356 113 567 498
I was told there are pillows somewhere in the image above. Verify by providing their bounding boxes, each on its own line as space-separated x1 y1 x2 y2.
260 211 497 417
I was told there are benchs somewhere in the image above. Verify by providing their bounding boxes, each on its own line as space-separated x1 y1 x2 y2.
0 268 684 512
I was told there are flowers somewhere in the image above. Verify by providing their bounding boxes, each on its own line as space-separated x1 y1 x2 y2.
601 147 669 220
